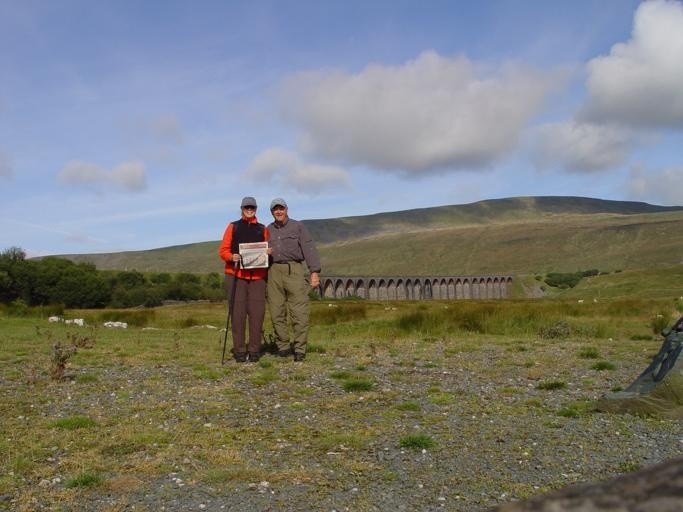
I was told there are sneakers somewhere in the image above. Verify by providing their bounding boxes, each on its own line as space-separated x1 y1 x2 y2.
280 349 290 356
234 352 247 362
249 352 259 362
294 352 305 362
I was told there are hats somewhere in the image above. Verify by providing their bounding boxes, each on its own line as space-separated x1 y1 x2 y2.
241 197 257 207
270 198 287 210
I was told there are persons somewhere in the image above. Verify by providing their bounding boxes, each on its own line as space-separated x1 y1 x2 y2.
265 197 322 362
217 196 270 362
